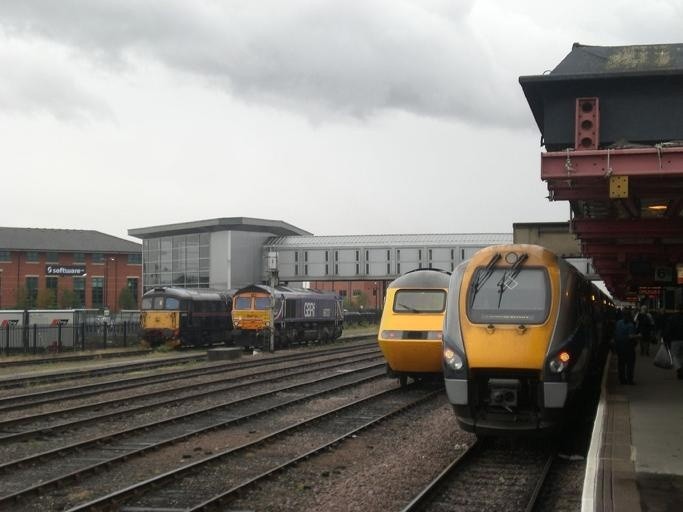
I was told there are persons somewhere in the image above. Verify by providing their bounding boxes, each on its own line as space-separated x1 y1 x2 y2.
614 304 682 386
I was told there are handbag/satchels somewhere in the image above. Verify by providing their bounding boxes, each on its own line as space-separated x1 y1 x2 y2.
651 336 675 370
606 332 630 354
645 334 659 345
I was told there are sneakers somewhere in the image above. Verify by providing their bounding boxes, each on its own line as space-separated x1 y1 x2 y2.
619 379 637 385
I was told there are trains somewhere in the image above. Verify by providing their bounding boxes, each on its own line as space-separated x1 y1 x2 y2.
227 283 344 350
439 243 618 437
137 282 233 349
376 266 451 389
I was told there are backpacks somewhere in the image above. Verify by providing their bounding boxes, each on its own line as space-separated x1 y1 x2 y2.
636 311 652 337
